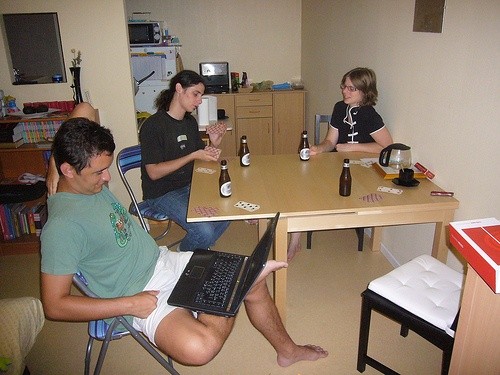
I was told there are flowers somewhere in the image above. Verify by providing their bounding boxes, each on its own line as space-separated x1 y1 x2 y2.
71 49 81 67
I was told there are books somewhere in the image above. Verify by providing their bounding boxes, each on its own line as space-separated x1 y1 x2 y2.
0 119 66 241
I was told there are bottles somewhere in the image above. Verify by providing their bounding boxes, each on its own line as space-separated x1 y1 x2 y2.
300 131 310 161
240 135 250 167
219 159 232 198
339 159 352 196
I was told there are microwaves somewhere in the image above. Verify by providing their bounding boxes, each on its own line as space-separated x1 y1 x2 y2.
128 21 162 46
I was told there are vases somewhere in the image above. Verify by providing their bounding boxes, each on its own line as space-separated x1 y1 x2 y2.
69 67 83 104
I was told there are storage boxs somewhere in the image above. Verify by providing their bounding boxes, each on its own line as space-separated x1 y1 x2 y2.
450 217 500 293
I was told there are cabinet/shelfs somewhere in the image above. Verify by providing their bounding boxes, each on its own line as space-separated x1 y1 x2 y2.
0 109 108 261
212 91 309 157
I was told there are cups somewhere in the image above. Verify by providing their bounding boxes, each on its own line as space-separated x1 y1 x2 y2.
400 168 415 183
218 109 225 118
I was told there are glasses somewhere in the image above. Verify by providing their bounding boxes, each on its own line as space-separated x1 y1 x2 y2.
339 83 358 91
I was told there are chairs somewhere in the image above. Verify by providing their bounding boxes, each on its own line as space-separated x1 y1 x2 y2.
72 272 181 375
116 144 184 249
356 257 467 375
306 113 364 251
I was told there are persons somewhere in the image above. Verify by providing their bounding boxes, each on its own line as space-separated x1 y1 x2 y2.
139 70 229 252
37 102 328 367
243 67 392 257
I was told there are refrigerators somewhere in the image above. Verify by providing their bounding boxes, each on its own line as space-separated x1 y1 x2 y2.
129 45 177 117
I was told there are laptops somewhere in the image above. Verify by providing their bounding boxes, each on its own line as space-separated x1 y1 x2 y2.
167 212 281 318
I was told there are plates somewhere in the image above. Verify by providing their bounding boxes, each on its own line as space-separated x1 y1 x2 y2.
218 116 228 119
392 178 420 186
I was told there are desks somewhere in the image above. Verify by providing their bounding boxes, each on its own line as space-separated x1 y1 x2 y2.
186 151 459 329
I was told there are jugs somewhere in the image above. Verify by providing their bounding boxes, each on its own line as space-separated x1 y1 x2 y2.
379 143 412 169
